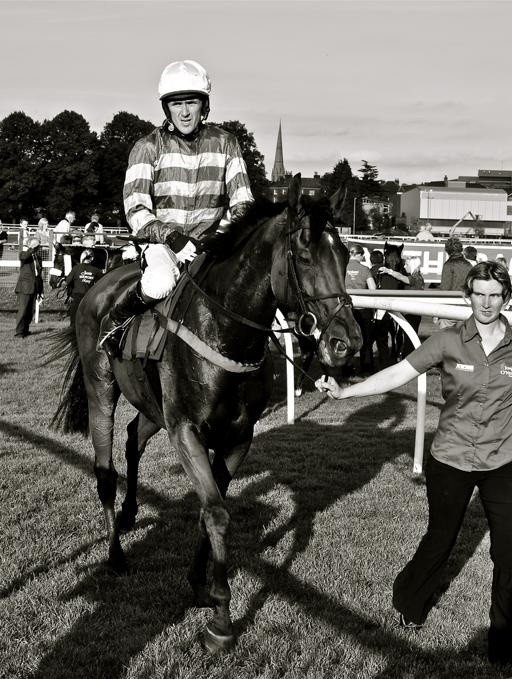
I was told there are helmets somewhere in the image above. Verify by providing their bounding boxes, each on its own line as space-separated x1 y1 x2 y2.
158 58 210 118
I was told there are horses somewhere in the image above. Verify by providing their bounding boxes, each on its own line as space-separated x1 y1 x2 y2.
47 240 133 291
35 164 364 658
381 240 412 363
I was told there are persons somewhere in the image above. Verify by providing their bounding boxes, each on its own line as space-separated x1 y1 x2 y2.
14 211 104 346
0 220 8 260
313 260 511 665
414 225 425 240
99 60 256 360
432 236 511 382
419 223 435 242
344 244 426 373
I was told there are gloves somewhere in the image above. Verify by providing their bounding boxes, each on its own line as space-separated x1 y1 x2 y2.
167 231 200 264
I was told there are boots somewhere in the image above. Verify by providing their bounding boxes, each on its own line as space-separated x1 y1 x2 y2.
99 281 149 359
